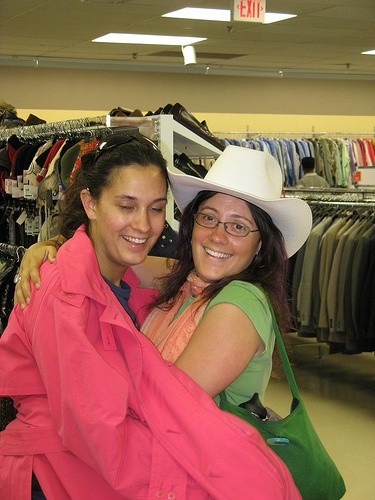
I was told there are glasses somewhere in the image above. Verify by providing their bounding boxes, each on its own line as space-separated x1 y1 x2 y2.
90 134 154 162
191 209 259 236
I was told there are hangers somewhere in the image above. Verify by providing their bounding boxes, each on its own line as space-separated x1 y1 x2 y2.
282 185 375 225
0 243 28 264
0 116 131 141
216 130 375 141
0 198 55 220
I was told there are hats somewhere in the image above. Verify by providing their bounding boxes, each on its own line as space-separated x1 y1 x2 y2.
164 145 312 264
0 134 98 200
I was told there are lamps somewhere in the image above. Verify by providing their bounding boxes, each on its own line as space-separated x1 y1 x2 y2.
181 46 196 67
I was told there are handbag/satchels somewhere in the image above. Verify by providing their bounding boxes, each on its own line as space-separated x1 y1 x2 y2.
200 280 345 500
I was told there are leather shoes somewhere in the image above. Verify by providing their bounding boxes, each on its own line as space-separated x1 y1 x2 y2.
173 151 207 179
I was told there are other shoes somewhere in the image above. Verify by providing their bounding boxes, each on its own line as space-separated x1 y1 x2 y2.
109 102 225 151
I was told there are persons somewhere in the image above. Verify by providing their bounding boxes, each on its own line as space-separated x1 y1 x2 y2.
13 144 312 500
296 157 330 188
0 135 171 500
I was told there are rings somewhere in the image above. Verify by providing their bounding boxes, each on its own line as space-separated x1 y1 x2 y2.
14 274 21 283
